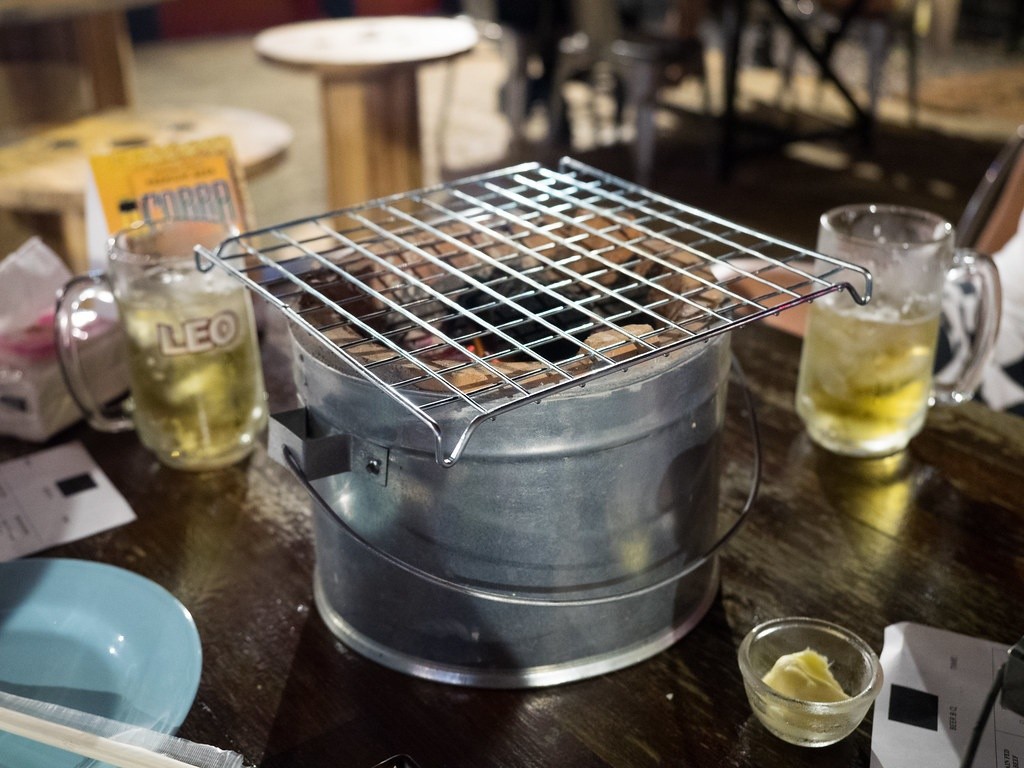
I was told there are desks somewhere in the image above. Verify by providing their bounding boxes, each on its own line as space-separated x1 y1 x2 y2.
251 14 482 230
0 316 1024 768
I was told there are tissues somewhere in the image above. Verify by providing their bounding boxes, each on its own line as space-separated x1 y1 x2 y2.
0 232 136 445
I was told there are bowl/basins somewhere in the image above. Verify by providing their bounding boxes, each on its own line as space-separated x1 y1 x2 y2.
737 614 885 748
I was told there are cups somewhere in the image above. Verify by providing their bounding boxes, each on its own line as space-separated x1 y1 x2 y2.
51 214 271 474
792 204 1004 462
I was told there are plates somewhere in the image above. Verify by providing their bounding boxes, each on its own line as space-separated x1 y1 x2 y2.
0 558 203 767
252 14 479 66
2 105 294 198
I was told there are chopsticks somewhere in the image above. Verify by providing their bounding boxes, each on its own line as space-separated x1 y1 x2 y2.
0 708 197 767
